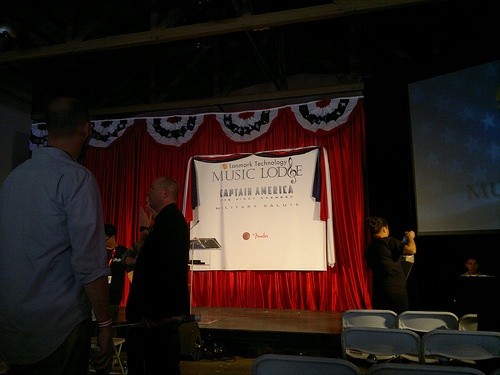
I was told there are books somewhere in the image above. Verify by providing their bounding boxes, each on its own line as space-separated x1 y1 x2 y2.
189 237 205 249
199 237 221 249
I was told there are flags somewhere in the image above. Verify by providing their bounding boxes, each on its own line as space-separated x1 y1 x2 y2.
464 107 500 182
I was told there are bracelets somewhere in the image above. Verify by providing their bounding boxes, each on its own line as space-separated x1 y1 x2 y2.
99 321 113 327
98 319 112 324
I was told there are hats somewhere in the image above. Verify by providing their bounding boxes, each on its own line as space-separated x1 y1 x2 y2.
140 226 149 232
104 223 117 236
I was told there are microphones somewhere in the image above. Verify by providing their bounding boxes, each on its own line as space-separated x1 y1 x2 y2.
190 220 199 230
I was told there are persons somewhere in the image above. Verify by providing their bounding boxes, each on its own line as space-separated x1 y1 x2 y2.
125 175 191 375
0 97 114 375
476 178 500 197
365 216 416 316
463 175 480 200
460 256 484 276
103 223 134 308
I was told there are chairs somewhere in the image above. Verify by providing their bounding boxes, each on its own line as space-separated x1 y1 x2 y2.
251 308 500 375
